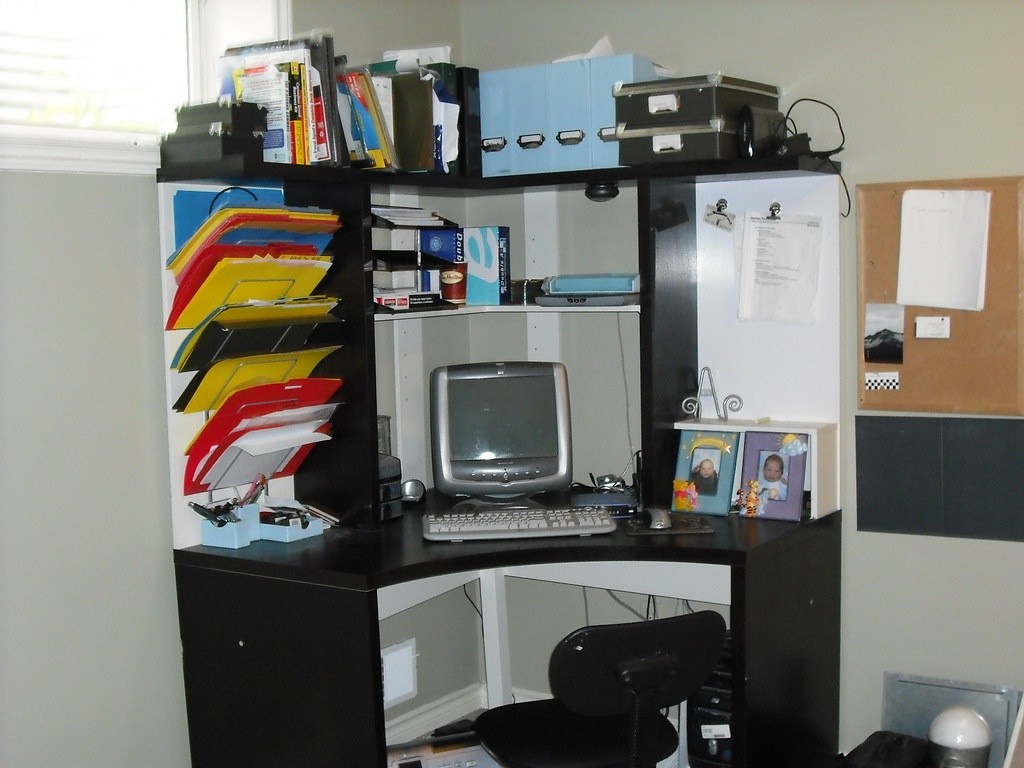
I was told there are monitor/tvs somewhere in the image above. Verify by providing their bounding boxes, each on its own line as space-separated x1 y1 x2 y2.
429 362 572 512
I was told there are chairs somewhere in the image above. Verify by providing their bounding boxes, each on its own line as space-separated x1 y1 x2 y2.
471 611 728 768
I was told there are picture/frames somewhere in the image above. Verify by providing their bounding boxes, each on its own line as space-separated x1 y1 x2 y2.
668 430 740 516
737 431 809 522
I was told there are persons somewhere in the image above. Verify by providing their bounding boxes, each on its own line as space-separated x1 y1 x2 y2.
691 459 718 496
757 453 787 500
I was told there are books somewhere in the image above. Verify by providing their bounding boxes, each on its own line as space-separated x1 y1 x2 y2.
217 34 399 171
372 207 443 226
549 274 639 294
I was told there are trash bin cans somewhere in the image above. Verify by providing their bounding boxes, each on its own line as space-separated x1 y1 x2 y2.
927 706 994 768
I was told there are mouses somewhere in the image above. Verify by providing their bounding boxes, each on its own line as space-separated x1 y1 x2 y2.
643 504 671 530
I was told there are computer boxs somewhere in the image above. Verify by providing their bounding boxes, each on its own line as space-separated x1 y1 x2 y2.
687 628 730 768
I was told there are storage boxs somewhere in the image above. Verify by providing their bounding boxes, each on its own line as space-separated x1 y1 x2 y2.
612 72 782 163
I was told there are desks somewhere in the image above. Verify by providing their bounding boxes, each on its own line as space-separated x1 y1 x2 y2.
173 481 843 768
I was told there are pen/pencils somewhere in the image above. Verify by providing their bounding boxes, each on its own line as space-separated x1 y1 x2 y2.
187 472 268 528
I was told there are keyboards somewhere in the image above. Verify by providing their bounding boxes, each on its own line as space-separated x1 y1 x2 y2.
422 504 617 542
